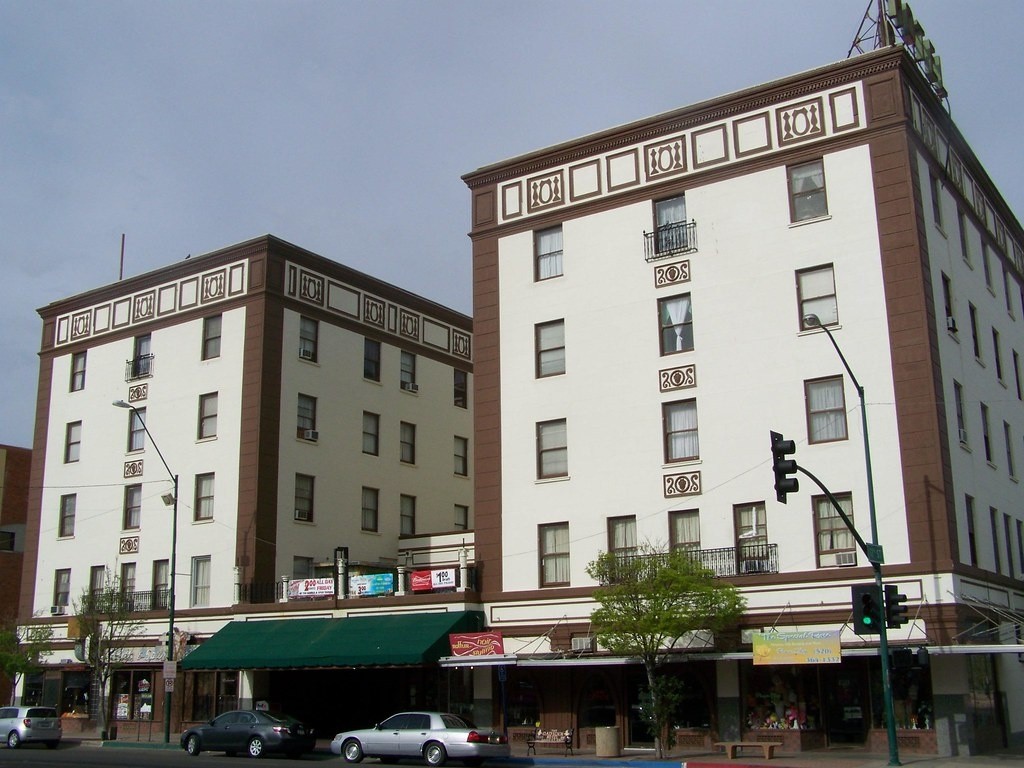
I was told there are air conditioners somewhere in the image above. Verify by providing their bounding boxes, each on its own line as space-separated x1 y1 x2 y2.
299 348 312 360
959 429 968 444
743 560 761 572
305 430 319 440
835 551 857 566
404 382 419 392
50 606 65 615
295 510 308 521
947 316 956 329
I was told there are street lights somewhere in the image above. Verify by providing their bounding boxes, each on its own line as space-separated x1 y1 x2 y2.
112 399 179 742
801 313 904 768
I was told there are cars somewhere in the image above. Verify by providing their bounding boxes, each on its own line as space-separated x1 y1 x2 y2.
180 709 318 757
330 711 511 766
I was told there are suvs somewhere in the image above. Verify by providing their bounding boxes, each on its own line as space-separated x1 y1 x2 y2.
0 705 63 750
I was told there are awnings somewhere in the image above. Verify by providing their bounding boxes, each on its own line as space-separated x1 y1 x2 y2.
175 611 465 673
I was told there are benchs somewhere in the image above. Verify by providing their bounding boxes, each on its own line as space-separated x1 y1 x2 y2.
527 727 575 758
714 742 784 760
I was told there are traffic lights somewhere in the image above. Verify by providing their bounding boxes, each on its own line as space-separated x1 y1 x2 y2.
850 583 882 634
885 583 908 630
770 429 800 503
